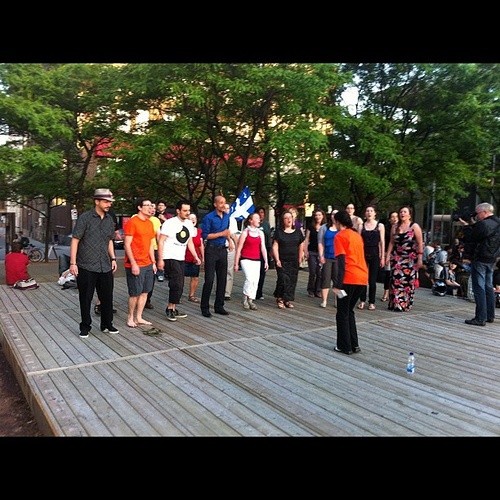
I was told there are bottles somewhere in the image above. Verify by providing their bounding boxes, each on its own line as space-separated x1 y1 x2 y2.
406 352 415 375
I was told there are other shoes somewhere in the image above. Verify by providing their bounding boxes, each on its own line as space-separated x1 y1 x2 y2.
316 291 322 298
333 345 360 354
174 309 187 318
144 303 153 309
241 298 250 310
249 300 257 310
94 304 100 314
214 307 229 315
225 296 231 300
381 296 387 301
488 317 494 322
464 318 487 326
165 307 177 321
142 328 161 337
113 310 116 313
201 309 212 317
321 302 327 308
309 291 314 298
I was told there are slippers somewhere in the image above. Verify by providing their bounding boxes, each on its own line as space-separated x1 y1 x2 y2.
369 304 376 310
357 302 364 309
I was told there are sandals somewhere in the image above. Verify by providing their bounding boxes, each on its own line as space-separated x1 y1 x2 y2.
283 301 294 308
188 295 200 302
276 297 286 308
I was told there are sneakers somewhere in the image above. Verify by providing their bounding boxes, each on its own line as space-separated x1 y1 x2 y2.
101 324 119 334
78 329 88 338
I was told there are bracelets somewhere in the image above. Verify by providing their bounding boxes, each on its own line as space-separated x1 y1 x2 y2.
416 252 423 255
70 264 77 265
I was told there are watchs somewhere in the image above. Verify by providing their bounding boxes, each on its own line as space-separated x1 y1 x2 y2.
111 257 116 261
233 264 237 266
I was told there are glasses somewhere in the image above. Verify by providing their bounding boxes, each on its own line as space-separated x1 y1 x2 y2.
143 205 153 208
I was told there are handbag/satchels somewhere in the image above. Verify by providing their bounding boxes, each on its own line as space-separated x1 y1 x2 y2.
14 278 38 290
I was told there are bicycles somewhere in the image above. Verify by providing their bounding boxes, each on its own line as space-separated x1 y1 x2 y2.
22 243 46 262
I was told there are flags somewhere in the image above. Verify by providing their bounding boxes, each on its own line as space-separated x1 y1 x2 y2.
228 187 255 222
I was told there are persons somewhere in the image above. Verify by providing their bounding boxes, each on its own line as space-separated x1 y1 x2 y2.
319 202 385 310
5 242 31 285
200 196 230 318
304 208 326 298
459 202 500 326
254 207 268 300
271 206 305 308
386 206 423 312
415 233 500 308
124 199 205 328
223 205 237 300
70 188 119 338
234 213 269 310
13 230 29 254
331 211 368 354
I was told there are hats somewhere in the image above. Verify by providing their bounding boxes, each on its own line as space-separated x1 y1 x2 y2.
92 188 115 202
